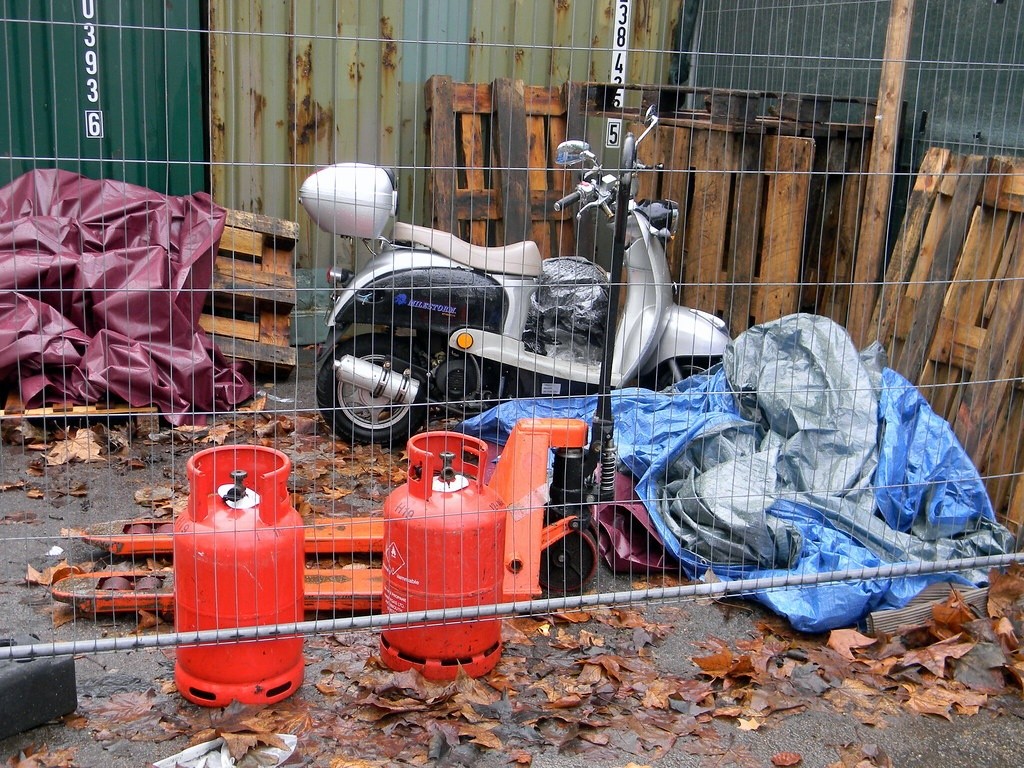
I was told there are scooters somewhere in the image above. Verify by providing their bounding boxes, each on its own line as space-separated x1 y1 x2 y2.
298 101 734 443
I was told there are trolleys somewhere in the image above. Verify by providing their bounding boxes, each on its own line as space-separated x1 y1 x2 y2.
46 135 634 623
